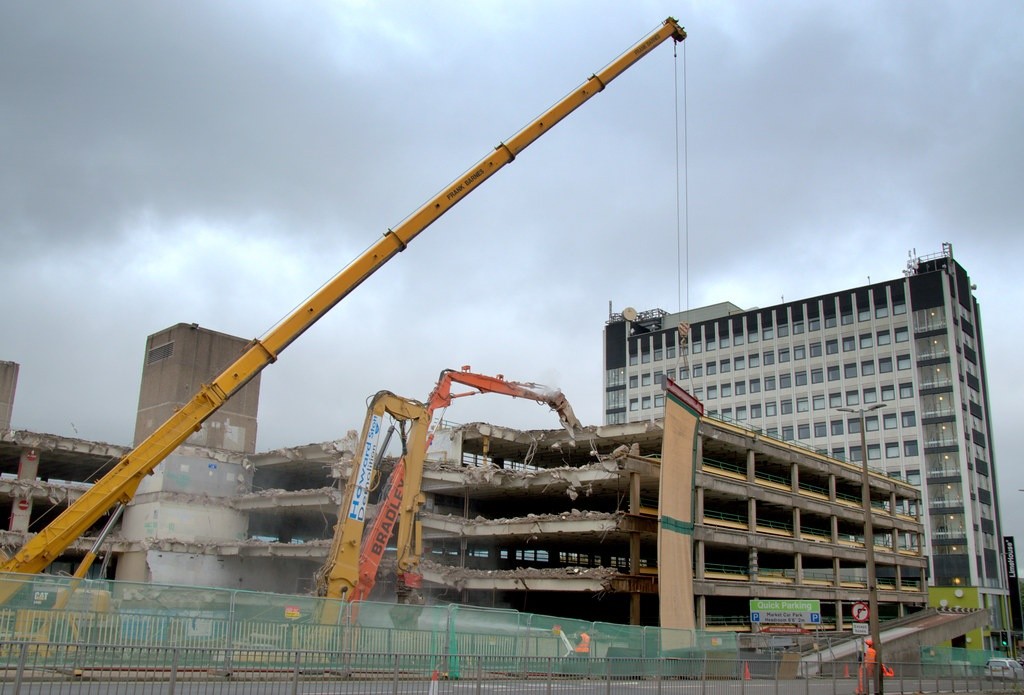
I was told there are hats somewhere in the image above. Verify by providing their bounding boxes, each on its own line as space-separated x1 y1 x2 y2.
865 639 872 645
579 626 586 631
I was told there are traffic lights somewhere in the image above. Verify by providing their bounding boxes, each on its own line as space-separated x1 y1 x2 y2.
1000 630 1008 646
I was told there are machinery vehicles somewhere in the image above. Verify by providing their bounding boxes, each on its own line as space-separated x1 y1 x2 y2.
0 16 686 664
207 365 584 669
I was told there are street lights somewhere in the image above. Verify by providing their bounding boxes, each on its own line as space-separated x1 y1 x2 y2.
836 403 889 694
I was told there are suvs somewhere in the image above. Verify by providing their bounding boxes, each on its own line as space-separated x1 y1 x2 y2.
983 657 1024 681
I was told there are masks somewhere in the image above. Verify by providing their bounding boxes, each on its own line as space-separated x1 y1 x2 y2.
865 643 869 648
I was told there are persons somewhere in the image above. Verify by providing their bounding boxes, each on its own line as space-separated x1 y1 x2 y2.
573 626 591 662
854 638 876 695
